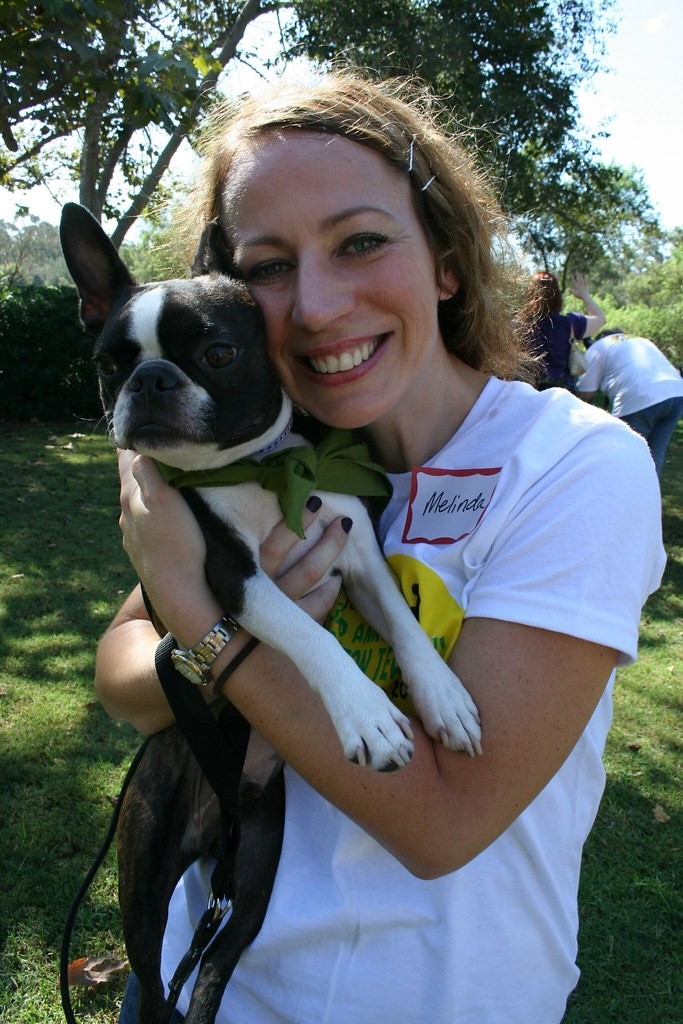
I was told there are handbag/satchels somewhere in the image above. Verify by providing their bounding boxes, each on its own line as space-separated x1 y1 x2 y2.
569 321 586 376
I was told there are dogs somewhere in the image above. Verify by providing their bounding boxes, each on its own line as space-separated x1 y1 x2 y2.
57 201 487 1024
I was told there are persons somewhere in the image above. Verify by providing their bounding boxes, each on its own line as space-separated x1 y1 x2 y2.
577 327 682 475
92 73 666 1023
521 272 605 393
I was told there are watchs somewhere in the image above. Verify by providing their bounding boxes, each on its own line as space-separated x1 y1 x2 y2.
171 617 239 686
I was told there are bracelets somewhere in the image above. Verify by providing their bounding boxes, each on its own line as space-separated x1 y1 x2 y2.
212 637 260 694
